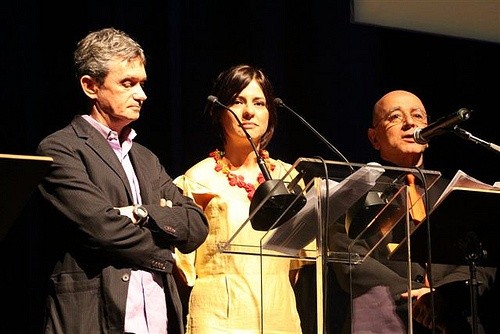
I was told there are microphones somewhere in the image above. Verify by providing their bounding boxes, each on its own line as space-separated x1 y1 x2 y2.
208 96 307 231
413 108 472 145
273 97 389 240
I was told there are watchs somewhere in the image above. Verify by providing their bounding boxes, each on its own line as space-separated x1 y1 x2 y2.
131 203 148 225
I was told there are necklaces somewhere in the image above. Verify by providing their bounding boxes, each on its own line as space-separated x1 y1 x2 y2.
211 148 277 200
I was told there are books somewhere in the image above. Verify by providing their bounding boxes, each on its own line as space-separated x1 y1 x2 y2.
387 169 499 267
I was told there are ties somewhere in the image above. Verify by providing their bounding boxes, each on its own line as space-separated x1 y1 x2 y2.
406 173 427 223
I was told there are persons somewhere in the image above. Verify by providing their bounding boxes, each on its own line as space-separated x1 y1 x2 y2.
333 89 500 334
173 65 308 334
34 27 210 333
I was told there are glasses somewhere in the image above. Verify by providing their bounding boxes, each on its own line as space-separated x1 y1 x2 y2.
375 109 429 128
